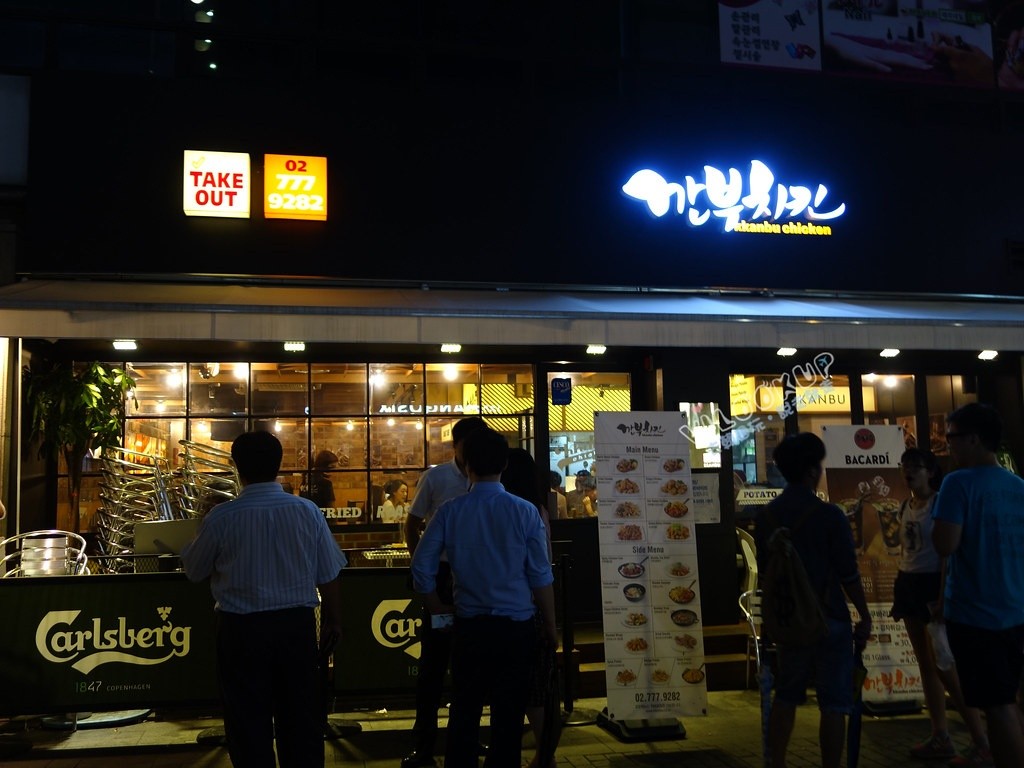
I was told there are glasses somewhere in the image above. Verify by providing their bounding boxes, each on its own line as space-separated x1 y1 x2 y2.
896 462 926 472
945 432 976 444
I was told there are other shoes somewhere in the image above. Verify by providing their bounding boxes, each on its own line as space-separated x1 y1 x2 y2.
523 753 557 768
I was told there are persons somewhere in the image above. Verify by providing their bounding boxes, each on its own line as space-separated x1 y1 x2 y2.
931 403 1024 768
890 447 988 762
299 448 598 523
180 430 352 768
403 417 560 768
753 432 872 768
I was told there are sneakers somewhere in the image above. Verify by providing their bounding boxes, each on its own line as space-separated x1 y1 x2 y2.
948 742 994 768
910 736 957 758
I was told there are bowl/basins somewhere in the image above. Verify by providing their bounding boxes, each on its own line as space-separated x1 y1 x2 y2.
623 583 647 602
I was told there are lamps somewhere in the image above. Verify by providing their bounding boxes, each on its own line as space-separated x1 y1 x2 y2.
198 366 213 380
409 391 415 402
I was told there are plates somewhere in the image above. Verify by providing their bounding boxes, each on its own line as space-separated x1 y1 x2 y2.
664 523 694 542
615 678 638 686
658 478 692 499
613 458 641 474
658 457 690 475
671 642 699 652
647 675 671 685
624 645 653 654
665 568 696 578
614 524 646 543
621 617 650 628
610 478 645 520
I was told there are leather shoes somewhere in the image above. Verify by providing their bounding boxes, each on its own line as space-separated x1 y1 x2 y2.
401 747 436 768
478 742 489 754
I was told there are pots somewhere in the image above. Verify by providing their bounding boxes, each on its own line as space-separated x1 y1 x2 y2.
664 498 691 518
668 580 698 603
681 662 705 685
670 609 700 628
618 556 649 579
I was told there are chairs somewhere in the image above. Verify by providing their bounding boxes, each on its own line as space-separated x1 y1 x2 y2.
0 530 91 731
738 589 777 691
94 440 240 574
